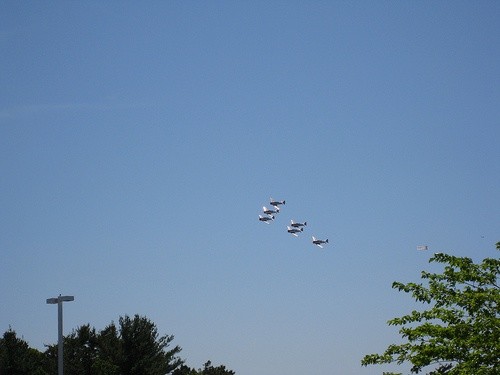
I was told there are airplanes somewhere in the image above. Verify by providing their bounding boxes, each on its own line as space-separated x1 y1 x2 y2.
263 207 279 219
257 215 273 224
287 226 303 238
311 235 329 249
270 197 286 211
291 219 307 229
